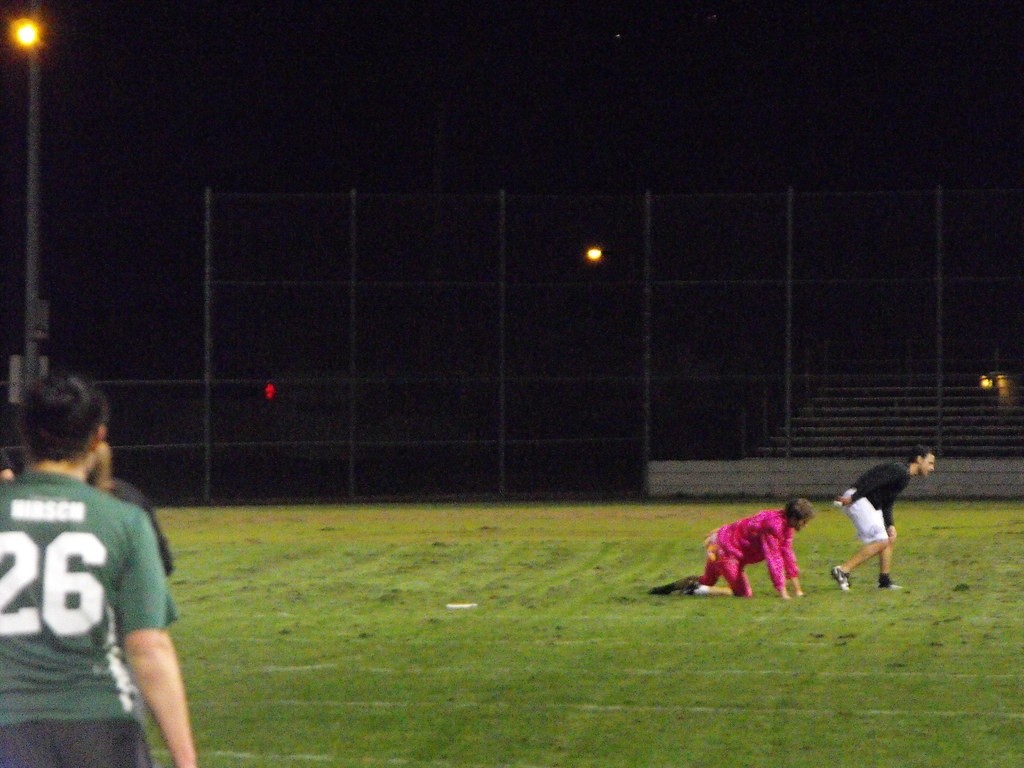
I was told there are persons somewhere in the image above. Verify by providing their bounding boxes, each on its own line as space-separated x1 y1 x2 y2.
831 444 937 591
648 496 816 603
0 376 200 768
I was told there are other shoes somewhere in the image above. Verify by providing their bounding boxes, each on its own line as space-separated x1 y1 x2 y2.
649 575 699 596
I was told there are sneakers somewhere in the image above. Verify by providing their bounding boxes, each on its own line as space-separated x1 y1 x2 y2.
878 585 901 589
831 565 850 592
